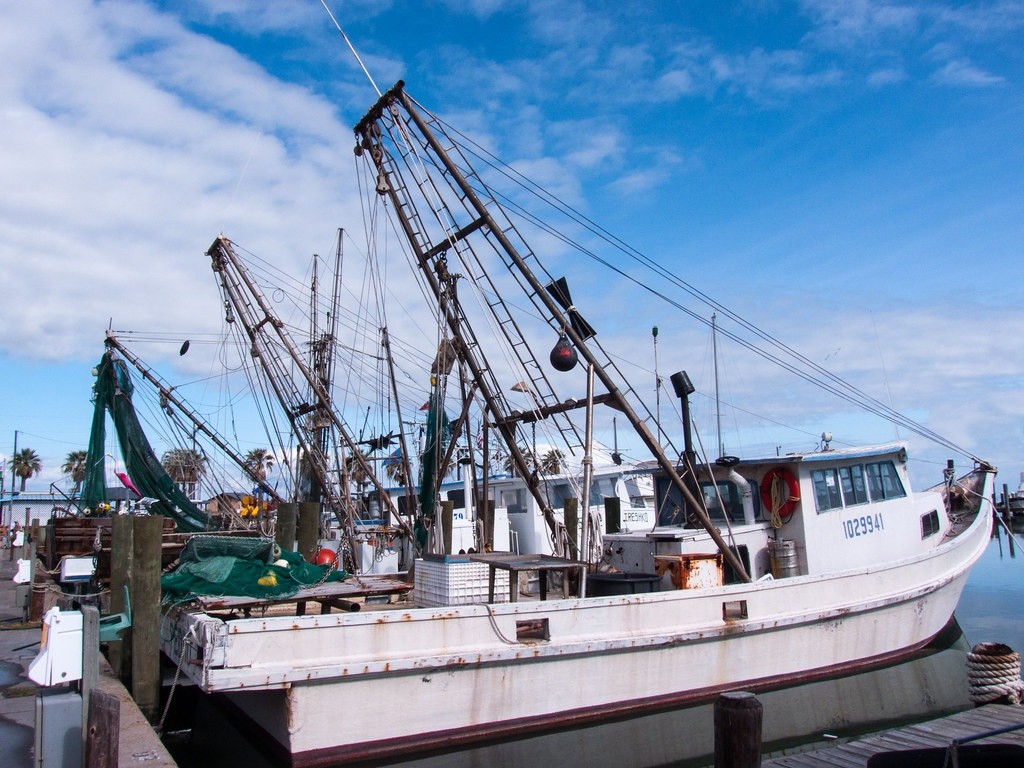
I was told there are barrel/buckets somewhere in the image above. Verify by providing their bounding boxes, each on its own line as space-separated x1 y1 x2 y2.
768 539 801 580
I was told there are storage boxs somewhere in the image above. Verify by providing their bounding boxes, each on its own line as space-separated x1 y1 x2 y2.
414 558 510 608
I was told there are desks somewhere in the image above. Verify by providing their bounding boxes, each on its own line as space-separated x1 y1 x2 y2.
469 553 587 603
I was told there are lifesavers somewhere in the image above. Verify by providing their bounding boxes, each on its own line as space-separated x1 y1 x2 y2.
760 466 801 518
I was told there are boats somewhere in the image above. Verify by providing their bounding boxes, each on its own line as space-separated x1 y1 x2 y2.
156 611 978 767
58 1 1001 767
1009 472 1024 516
